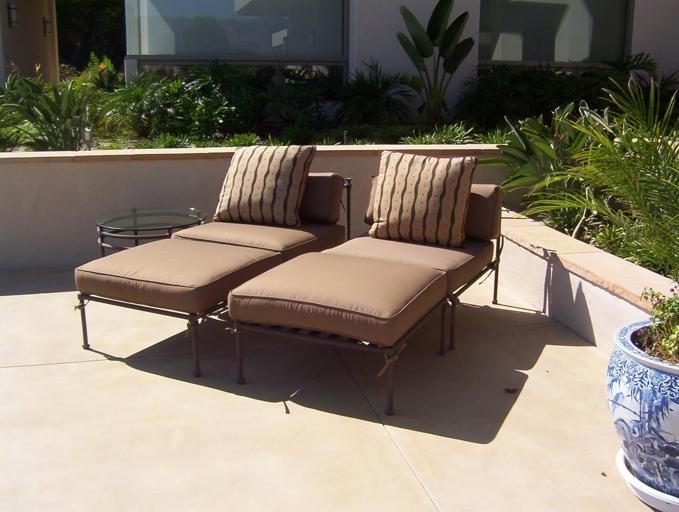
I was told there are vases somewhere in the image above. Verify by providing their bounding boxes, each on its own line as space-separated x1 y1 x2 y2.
607 319 678 500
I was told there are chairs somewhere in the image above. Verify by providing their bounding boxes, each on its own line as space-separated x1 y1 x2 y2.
227 182 502 417
74 171 352 376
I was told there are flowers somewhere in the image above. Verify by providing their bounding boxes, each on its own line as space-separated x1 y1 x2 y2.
636 284 678 362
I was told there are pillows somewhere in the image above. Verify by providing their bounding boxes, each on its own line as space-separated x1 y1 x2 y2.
212 142 478 251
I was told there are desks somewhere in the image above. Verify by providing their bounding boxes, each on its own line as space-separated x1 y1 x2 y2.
95 207 208 262
